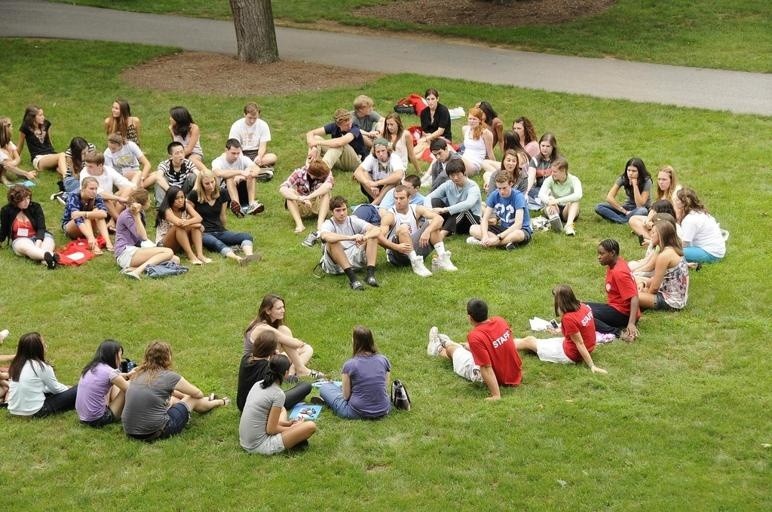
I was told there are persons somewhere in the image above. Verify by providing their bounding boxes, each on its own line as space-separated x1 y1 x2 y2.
236 330 312 412
515 284 608 374
580 238 642 343
244 295 326 383
426 297 523 401
121 340 230 443
318 324 393 420
6 332 78 418
0 329 17 409
75 339 180 428
594 157 727 310
1 97 277 282
279 86 582 290
239 353 317 455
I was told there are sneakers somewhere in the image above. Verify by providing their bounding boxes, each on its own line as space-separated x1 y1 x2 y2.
230 200 264 217
427 326 451 356
44 252 58 269
411 255 432 277
302 231 317 247
564 225 575 236
438 251 458 271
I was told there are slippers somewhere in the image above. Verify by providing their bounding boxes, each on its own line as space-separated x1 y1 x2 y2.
349 276 378 290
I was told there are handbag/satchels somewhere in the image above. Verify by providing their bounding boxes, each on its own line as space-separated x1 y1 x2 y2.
391 380 410 411
